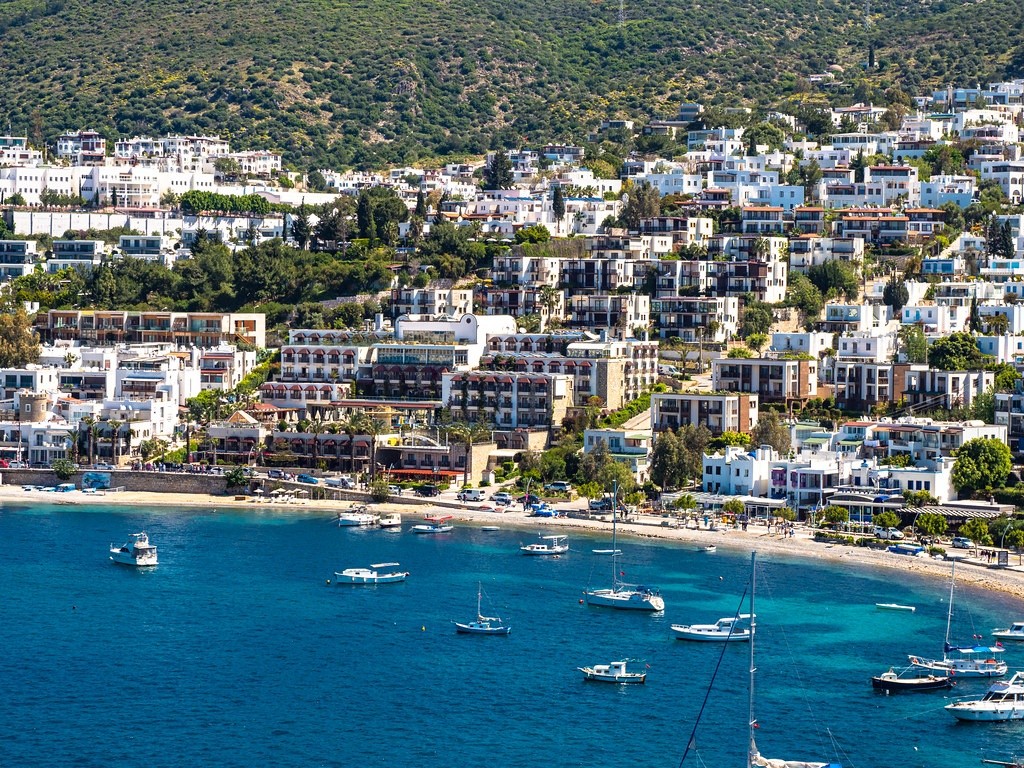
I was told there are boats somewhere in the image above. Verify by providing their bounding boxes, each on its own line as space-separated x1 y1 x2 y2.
108 529 159 566
333 561 406 582
592 549 622 556
519 534 570 556
576 661 647 684
339 502 453 533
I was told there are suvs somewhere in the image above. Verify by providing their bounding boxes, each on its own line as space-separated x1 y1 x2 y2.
545 480 572 494
416 484 438 497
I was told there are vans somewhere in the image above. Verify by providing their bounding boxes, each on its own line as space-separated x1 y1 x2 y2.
457 489 486 502
873 525 904 540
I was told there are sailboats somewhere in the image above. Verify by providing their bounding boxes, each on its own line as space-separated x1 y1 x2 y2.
581 479 665 613
678 550 855 768
450 579 512 635
871 558 1024 724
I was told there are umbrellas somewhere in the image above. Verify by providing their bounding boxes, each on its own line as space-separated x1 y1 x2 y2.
253 488 264 496
270 488 309 499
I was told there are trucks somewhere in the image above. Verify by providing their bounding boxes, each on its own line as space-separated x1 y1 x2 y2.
324 476 355 489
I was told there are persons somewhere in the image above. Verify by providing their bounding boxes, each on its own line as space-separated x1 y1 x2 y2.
523 500 529 511
768 521 771 533
980 549 996 563
153 461 166 472
920 538 927 546
621 509 628 517
293 473 295 481
704 516 708 526
789 523 794 528
217 466 220 474
695 517 698 522
463 493 466 503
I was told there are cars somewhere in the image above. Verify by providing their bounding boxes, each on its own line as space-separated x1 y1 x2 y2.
269 469 285 478
92 462 117 470
0 456 51 469
131 462 261 476
490 492 513 501
588 497 620 510
952 536 975 549
297 473 318 484
388 484 403 496
516 494 541 504
